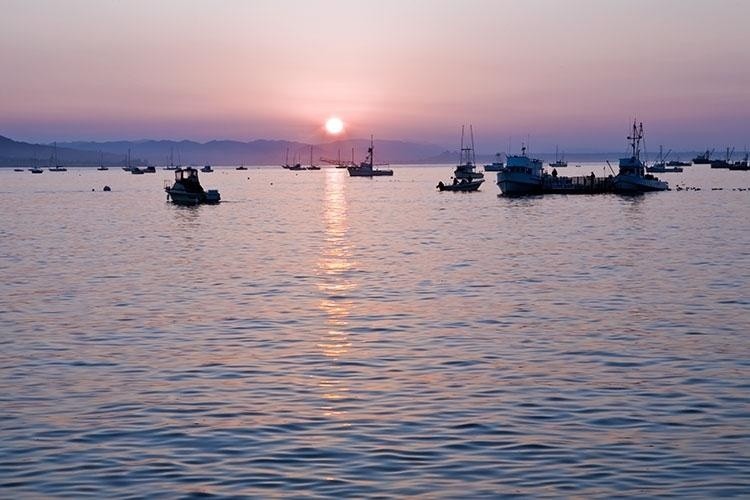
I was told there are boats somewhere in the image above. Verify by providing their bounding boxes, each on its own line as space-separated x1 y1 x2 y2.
437 179 486 192
483 162 505 171
163 166 223 207
200 165 213 172
497 121 667 200
235 166 248 170
646 144 750 173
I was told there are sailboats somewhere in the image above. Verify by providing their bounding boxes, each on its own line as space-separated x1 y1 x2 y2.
280 145 320 171
122 148 156 175
96 152 109 172
335 136 393 176
454 124 484 178
164 148 181 171
14 140 69 175
